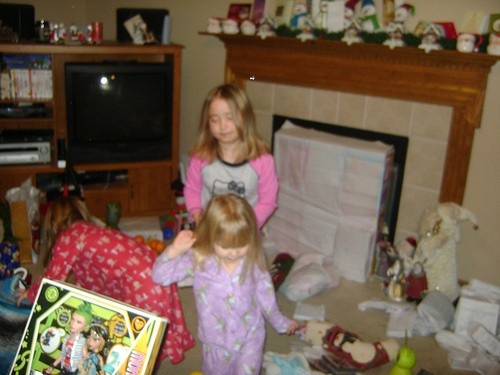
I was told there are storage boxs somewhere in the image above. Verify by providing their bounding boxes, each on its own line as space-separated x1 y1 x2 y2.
117 217 163 241
453 296 500 337
262 121 395 284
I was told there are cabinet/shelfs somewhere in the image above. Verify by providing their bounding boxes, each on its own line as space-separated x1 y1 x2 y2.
0 43 186 221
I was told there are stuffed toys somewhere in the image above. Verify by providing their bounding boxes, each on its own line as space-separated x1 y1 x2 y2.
382 200 479 309
305 320 400 371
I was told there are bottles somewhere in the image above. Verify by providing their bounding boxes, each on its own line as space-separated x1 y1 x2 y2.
35 20 67 44
69 22 81 41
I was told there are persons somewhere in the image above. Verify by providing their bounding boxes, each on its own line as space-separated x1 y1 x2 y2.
152 192 299 375
15 162 195 375
183 83 279 236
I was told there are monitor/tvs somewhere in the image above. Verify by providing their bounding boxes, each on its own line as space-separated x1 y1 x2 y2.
64 61 174 165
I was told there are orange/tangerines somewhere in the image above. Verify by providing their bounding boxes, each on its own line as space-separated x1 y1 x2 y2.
134 235 166 253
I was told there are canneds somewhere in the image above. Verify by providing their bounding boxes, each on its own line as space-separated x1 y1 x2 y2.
92 20 103 45
36 20 50 42
